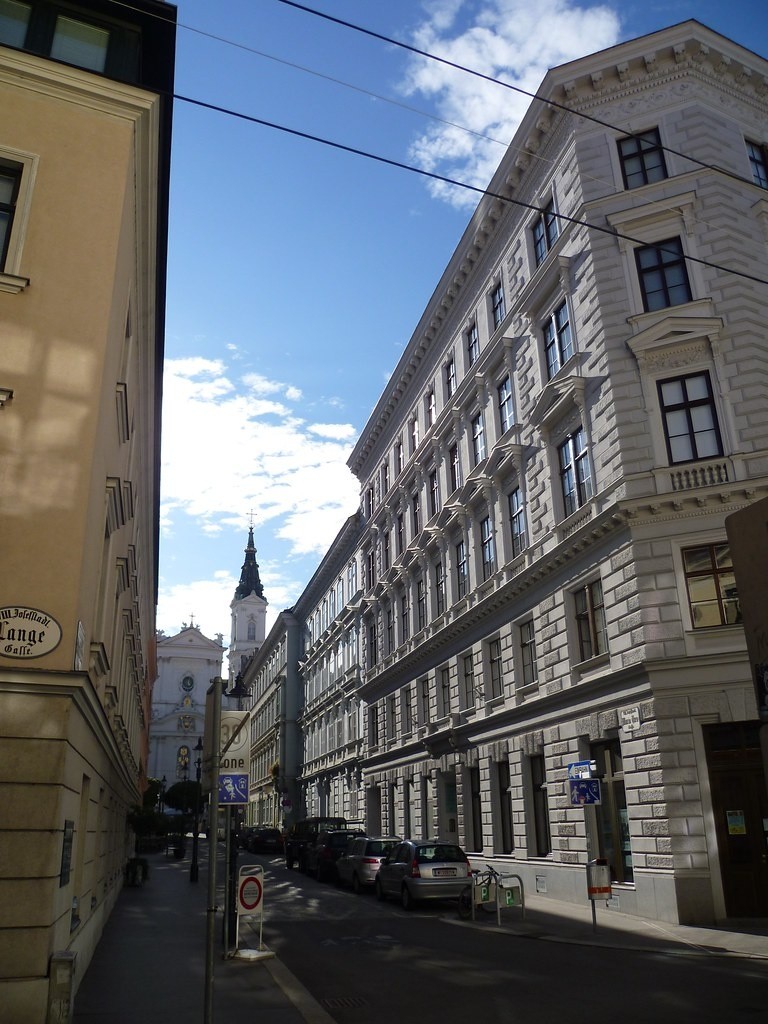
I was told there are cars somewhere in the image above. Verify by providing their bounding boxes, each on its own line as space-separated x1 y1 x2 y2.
238 827 284 854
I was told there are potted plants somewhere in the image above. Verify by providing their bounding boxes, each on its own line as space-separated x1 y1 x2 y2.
124 858 150 888
169 814 195 860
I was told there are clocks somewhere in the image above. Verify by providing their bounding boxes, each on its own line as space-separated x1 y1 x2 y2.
183 676 194 689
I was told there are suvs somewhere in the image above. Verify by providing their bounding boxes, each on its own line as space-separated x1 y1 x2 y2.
375 838 476 910
311 828 367 879
334 834 404 890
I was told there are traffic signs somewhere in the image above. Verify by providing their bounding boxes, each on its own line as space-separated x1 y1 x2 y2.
567 761 591 779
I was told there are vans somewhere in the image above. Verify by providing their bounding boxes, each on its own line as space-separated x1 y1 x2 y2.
286 816 347 870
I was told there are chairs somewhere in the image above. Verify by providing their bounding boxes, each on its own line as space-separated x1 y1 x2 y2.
419 856 426 860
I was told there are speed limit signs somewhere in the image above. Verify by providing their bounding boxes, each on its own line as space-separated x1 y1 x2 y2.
220 717 247 751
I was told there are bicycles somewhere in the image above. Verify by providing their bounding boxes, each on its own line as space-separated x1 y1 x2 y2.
459 864 504 918
